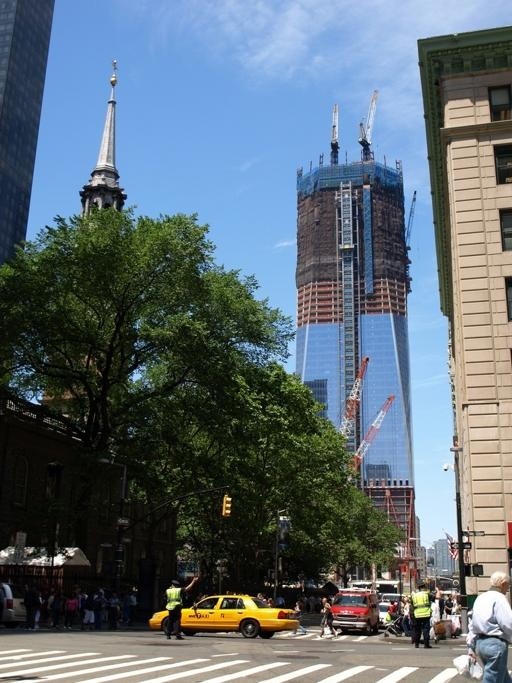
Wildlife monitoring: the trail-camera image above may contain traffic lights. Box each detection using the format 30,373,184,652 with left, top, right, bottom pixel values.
221,494,233,518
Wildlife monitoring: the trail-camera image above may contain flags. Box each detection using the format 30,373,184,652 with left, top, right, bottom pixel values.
447,535,459,560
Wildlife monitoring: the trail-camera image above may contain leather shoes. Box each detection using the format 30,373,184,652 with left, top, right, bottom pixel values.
167,636,184,640
416,645,431,648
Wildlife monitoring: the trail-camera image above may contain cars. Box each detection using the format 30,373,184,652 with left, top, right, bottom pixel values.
147,593,300,639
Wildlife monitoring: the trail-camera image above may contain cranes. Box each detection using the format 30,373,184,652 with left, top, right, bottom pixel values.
357,90,379,150
331,103,339,149
346,396,394,476
337,357,370,451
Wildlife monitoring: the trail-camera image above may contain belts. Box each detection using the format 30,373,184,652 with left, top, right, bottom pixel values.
480,634,500,639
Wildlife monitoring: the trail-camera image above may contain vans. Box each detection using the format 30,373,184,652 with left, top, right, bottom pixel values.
0,580,28,628
329,590,405,636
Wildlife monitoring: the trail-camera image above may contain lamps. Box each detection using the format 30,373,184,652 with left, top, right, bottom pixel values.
443,463,454,472
140,551,146,559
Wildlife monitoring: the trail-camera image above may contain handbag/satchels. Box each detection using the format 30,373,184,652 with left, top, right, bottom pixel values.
386,613,391,621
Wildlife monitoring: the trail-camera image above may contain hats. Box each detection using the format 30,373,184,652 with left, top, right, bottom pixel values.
172,580,179,587
415,581,425,588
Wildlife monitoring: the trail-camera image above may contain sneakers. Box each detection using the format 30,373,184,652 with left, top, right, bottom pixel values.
317,631,338,639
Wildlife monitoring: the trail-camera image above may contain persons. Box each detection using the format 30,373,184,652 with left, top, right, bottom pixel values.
193,590,285,608
465,570,512,683
319,597,339,638
0,584,5,625
387,579,462,649
297,593,335,614
291,601,308,634
23,584,138,630
164,576,199,640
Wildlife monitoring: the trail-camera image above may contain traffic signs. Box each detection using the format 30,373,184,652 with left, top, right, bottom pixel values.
451,542,471,549
